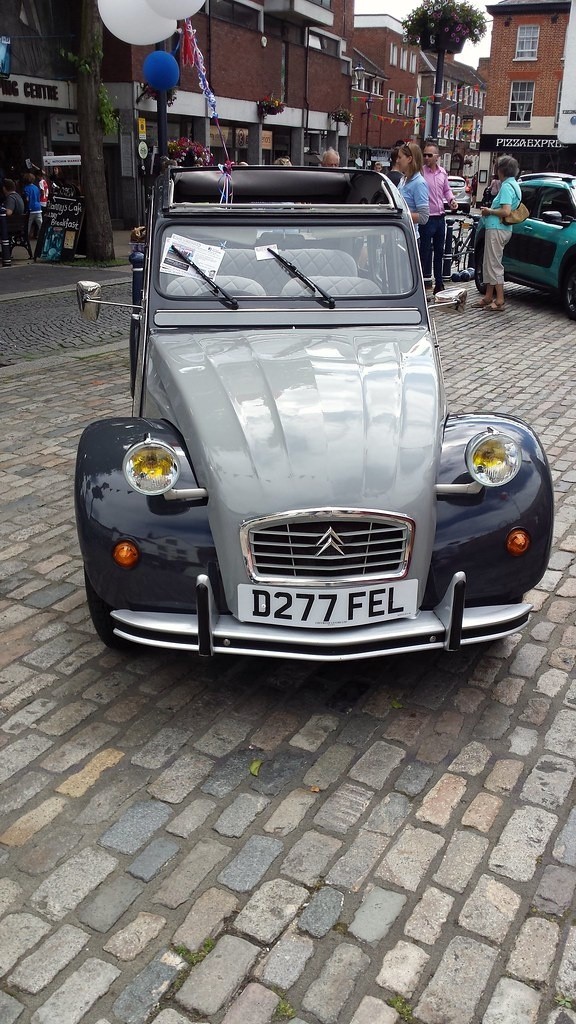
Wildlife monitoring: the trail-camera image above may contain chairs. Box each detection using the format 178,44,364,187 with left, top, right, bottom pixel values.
280,277,385,298
165,276,268,297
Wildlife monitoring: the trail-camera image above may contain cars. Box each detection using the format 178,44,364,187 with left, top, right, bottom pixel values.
74,163,554,660
443,176,471,213
474,172,576,321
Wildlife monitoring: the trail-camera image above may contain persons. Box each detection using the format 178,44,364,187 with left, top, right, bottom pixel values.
375,146,403,185
0,166,62,257
487,175,501,202
419,144,459,295
195,160,202,167
471,157,521,310
164,160,176,166
275,158,291,166
397,142,430,250
471,174,477,207
323,151,339,167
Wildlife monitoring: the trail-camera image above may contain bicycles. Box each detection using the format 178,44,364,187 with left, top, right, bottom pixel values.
451,212,481,271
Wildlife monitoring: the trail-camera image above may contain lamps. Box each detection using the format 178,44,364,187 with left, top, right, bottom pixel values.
362,92,374,114
349,61,365,89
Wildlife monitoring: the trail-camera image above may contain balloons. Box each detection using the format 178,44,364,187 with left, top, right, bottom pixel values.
143,51,180,91
98,0,177,45
147,0,205,20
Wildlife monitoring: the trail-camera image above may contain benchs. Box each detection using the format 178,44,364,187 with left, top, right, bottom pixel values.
5,210,35,261
162,250,357,281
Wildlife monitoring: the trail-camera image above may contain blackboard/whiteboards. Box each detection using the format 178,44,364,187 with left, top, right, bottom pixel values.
35,194,86,261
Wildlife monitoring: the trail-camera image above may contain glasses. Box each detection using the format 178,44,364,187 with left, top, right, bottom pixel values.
422,153,439,158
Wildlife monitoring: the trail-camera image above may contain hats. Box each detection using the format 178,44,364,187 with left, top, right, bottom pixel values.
397,140,404,145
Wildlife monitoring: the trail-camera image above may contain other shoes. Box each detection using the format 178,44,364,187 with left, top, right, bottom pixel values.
471,205,474,207
433,288,444,294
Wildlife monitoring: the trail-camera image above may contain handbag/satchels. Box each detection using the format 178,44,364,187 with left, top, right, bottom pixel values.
499,182,530,226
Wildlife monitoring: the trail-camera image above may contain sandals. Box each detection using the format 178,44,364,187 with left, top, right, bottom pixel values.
482,302,505,311
471,299,492,307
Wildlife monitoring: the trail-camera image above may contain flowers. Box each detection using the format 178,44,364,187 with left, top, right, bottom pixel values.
402,0,487,48
158,137,217,166
332,109,353,125
255,96,285,115
